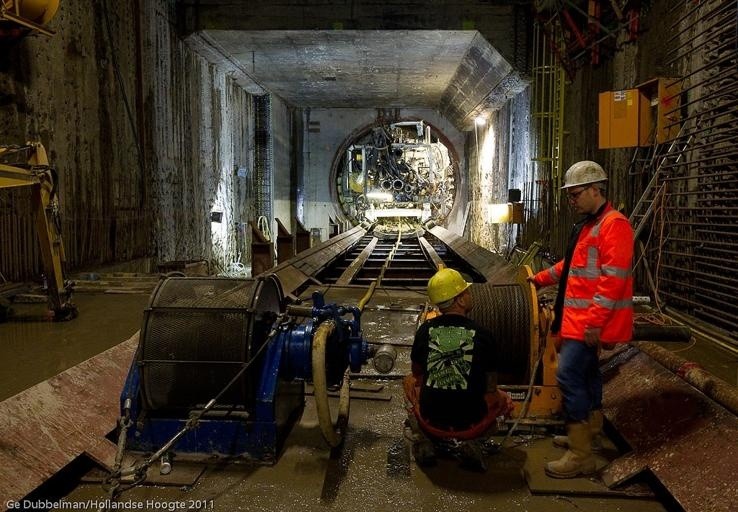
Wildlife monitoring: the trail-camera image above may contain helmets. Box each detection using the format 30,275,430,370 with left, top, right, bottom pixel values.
427,264,473,304
557,160,609,191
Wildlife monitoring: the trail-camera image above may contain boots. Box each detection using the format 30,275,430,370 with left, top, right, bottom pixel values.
408,415,438,465
544,422,597,479
552,409,604,453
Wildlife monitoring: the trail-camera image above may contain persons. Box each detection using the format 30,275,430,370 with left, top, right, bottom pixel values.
399,265,512,469
526,159,637,479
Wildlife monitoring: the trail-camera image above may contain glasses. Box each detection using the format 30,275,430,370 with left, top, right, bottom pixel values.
566,185,590,201
